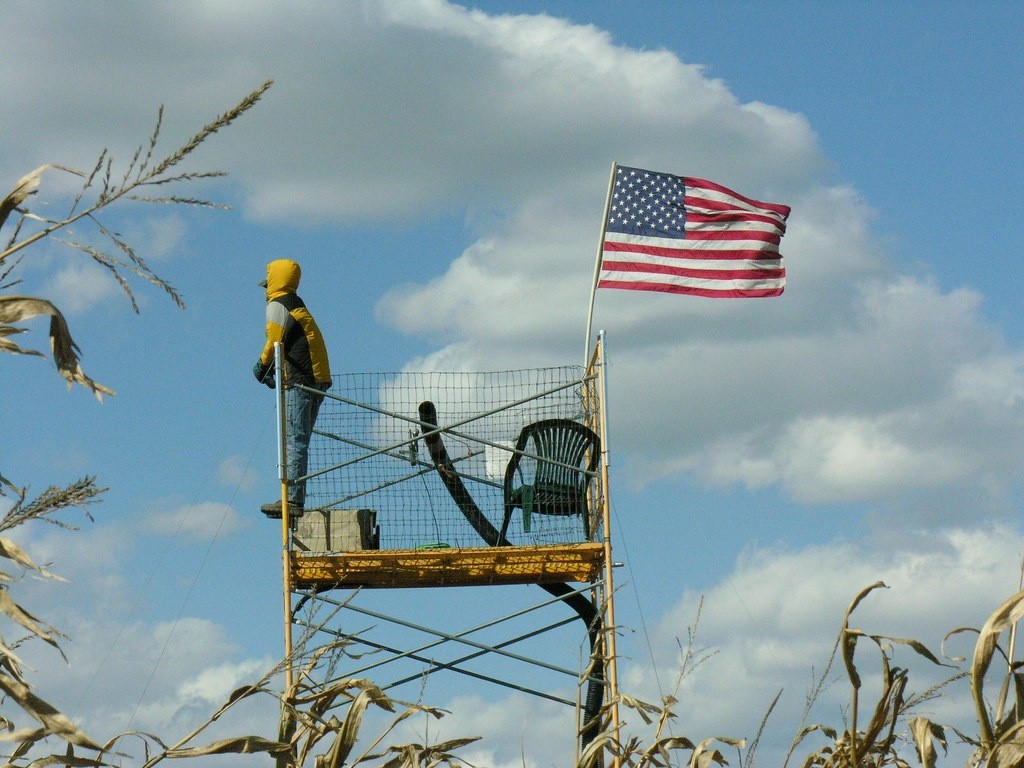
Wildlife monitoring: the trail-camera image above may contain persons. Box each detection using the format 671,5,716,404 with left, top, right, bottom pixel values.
254,259,332,519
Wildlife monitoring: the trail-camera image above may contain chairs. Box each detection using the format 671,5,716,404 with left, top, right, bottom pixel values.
494,418,603,546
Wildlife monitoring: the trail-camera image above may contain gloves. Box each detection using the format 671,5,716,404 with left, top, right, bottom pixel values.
265,370,276,389
253,357,269,383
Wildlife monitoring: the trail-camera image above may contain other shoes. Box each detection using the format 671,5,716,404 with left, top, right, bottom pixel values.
261,500,303,518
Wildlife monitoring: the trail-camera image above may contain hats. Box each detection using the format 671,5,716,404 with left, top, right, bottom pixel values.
257,279,268,286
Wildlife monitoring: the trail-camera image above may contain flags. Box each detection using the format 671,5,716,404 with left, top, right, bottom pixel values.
596,163,791,298
